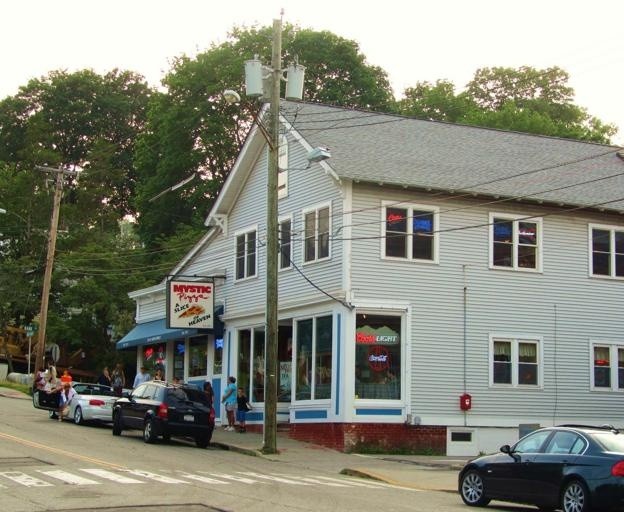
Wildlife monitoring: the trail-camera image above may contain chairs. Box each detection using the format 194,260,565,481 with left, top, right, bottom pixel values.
553,437,578,454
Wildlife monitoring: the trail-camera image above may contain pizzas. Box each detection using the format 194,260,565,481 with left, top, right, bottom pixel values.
179,304,205,318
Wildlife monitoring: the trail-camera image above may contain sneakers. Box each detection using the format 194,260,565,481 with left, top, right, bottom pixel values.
223,425,248,434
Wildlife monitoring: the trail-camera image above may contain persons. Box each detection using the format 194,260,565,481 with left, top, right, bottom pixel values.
31,360,255,434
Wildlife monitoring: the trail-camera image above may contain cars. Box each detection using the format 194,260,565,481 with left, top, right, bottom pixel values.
32,382,120,425
458,424,624,512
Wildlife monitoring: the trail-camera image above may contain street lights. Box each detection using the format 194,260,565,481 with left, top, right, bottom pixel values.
0,207,58,392
223,88,330,452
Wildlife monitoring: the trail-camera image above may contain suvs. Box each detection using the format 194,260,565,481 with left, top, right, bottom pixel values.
112,381,215,449
